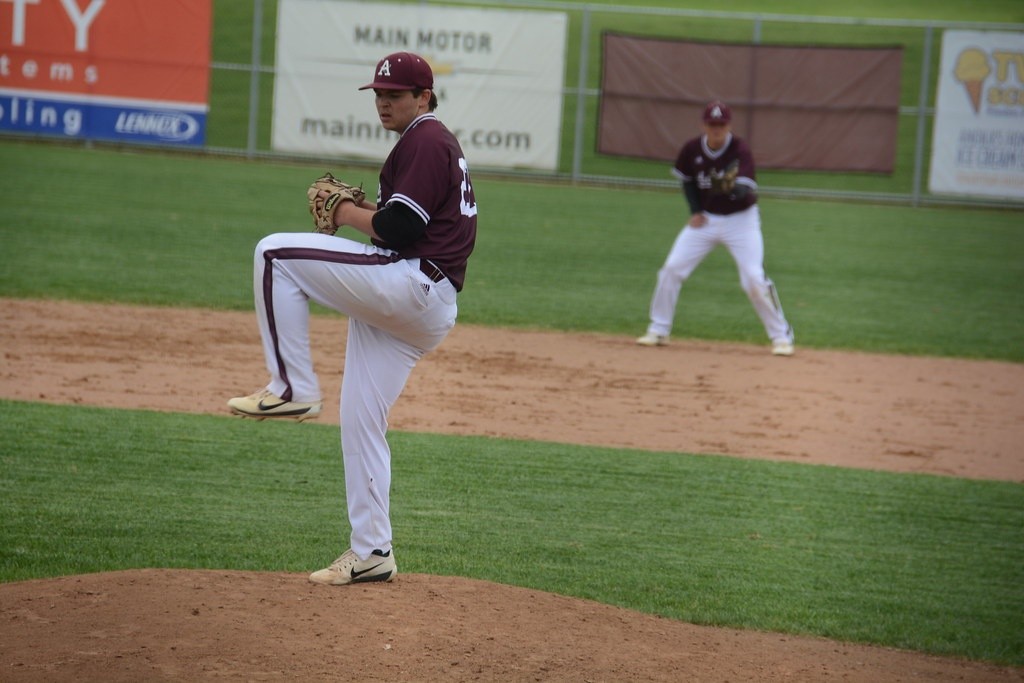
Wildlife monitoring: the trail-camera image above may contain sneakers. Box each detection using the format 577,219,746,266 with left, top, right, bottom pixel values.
772,342,793,356
226,388,320,423
635,332,670,346
309,549,397,586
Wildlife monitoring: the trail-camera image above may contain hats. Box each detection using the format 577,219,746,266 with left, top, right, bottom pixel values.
359,52,434,90
703,101,731,124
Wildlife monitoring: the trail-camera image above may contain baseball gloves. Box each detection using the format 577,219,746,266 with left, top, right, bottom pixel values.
308,176,365,235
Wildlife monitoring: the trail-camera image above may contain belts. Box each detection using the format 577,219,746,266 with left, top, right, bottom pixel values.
419,259,444,283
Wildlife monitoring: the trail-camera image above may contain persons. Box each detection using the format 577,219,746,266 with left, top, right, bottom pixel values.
636,102,796,355
228,51,478,585
